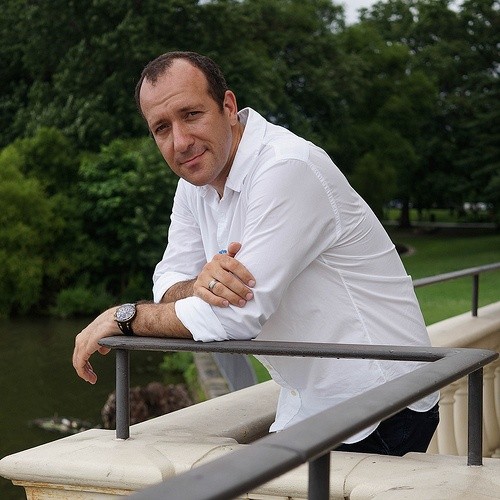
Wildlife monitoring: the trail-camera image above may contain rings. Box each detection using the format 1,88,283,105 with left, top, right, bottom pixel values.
208,279,218,291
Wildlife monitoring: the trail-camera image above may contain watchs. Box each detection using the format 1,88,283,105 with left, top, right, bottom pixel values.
114,303,138,337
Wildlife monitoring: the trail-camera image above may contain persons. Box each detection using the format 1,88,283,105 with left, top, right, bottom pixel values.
72,51,441,457
